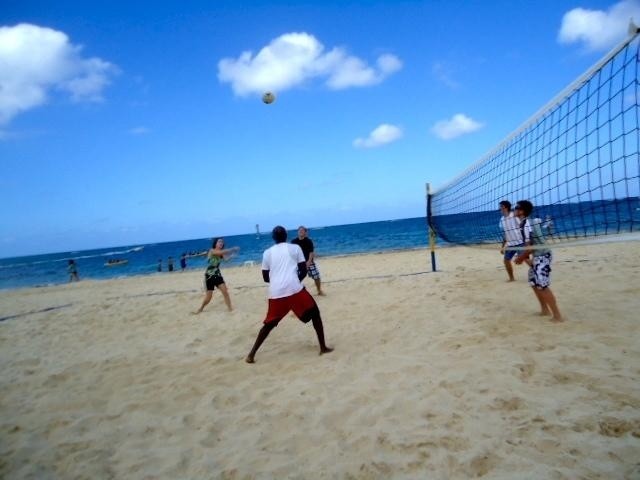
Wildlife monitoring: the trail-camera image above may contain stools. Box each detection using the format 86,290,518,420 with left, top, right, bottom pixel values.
243,260,256,270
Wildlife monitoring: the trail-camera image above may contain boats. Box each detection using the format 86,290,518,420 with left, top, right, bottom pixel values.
185,251,208,258
103,260,128,266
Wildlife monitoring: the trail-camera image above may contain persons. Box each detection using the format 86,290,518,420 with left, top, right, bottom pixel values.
189,237,242,316
290,226,327,297
496,200,532,283
243,227,335,364
66,260,79,283
167,257,173,272
180,253,187,273
156,259,162,272
511,200,565,322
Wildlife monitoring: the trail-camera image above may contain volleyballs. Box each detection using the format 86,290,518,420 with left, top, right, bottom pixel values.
261,91,275,104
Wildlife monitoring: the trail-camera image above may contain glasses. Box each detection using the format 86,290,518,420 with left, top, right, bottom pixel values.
514,206,522,210
